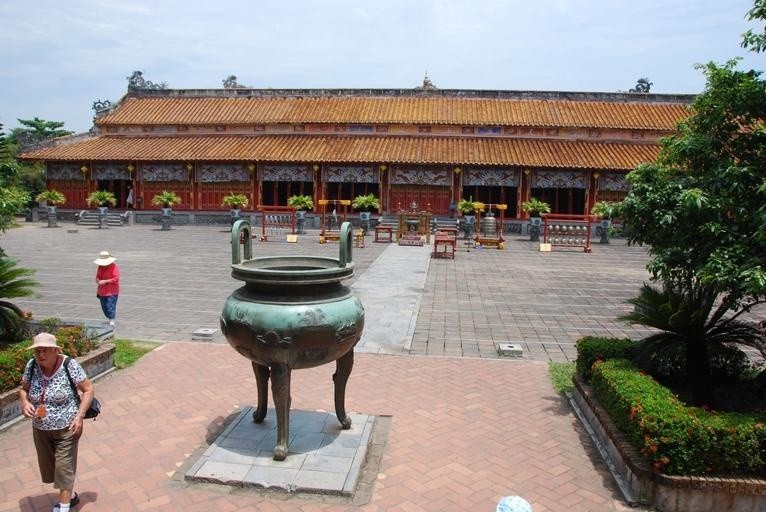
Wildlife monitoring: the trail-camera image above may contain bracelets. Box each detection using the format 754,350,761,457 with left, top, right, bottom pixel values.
76,408,86,418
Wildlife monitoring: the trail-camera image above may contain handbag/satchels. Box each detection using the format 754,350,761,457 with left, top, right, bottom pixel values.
84,397,101,419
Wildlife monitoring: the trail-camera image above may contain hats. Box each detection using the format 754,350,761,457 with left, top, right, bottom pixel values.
94,251,116,266
26,333,60,350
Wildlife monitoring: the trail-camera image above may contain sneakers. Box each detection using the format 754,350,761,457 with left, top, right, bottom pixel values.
109,319,115,325
52,492,79,508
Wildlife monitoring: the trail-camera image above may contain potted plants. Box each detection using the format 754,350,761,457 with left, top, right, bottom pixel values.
590,200,621,230
85,189,117,214
220,191,249,218
151,189,181,217
521,196,552,228
286,194,314,220
351,193,380,221
35,189,65,212
457,195,486,225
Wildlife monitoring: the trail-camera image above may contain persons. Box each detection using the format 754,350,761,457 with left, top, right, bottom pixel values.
93,250,120,326
17,331,95,511
125,184,133,210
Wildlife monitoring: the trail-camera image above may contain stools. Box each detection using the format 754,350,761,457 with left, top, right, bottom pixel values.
375,226,392,243
434,227,457,259
